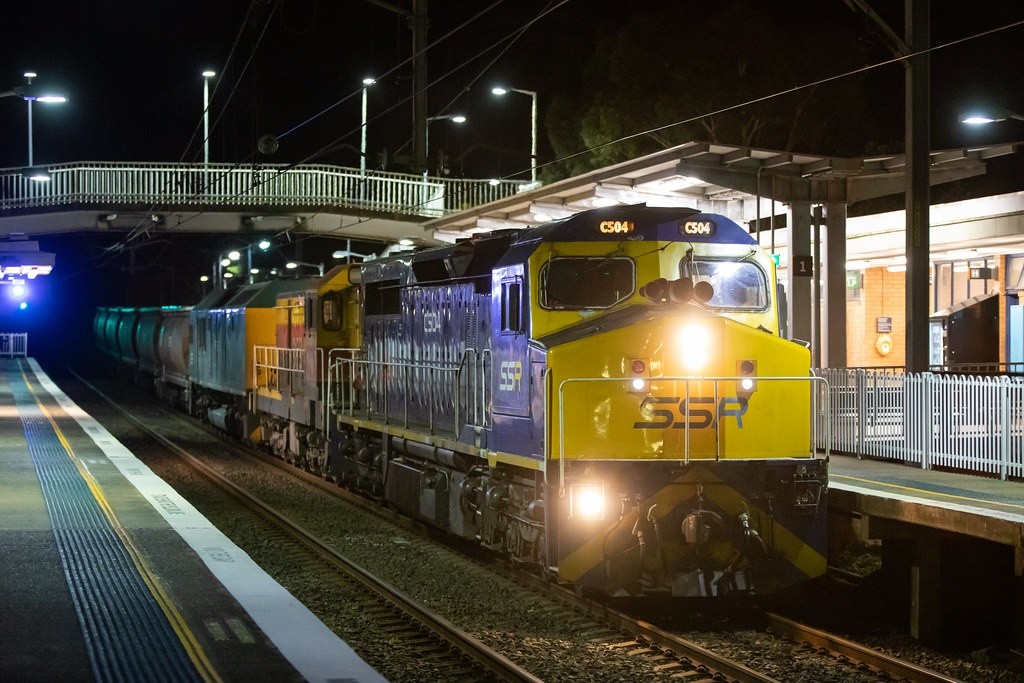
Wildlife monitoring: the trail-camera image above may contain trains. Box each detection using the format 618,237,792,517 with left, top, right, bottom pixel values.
83,202,830,600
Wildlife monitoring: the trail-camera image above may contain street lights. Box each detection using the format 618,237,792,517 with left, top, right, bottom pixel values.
286,262,323,277
200,70,217,204
491,84,538,187
421,115,466,214
362,77,376,206
24,70,37,204
